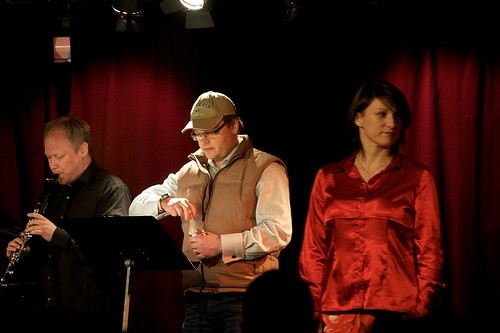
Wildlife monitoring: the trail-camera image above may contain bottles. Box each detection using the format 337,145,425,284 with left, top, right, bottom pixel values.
187,211,213,263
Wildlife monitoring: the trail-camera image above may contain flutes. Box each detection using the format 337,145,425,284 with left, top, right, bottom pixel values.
0,174,58,287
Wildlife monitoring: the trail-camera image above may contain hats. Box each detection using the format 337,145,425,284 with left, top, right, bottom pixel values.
181,90,237,133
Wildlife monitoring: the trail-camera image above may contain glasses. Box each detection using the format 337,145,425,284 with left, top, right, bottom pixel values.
190,117,231,141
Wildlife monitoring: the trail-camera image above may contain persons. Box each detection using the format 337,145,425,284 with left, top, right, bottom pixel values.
295,79,446,333
5,115,133,333
128,90,292,333
